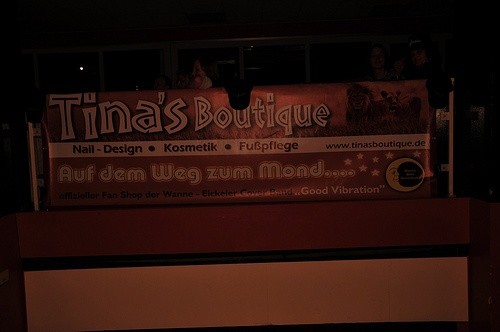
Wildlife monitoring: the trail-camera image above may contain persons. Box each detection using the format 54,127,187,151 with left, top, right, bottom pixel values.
369,43,390,81
175,69,190,88
154,75,172,90
190,57,212,88
188,75,203,89
387,55,405,81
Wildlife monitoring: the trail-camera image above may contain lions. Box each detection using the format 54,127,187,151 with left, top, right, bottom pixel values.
343,84,387,132
381,89,422,128
